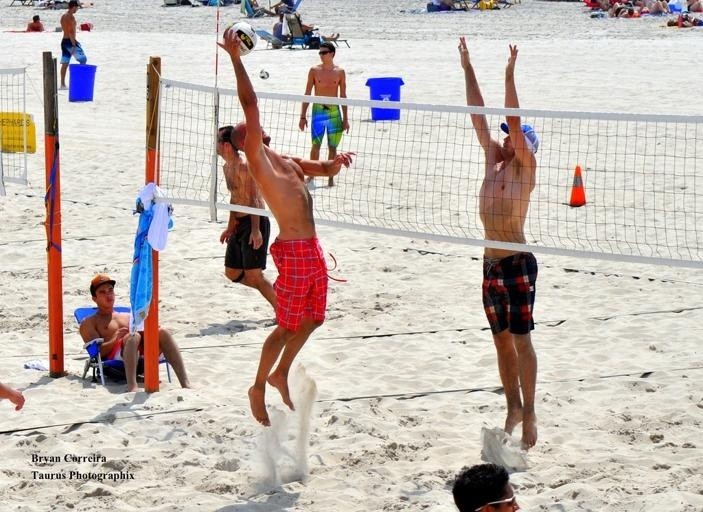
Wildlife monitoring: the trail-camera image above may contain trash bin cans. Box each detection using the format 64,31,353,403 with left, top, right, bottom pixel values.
68,64,96,103
365,78,405,122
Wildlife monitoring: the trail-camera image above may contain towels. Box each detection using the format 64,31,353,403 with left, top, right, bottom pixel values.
140,182,169,250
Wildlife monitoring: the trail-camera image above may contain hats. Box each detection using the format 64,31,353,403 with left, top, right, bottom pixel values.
67,0,85,10
500,121,539,156
89,275,117,292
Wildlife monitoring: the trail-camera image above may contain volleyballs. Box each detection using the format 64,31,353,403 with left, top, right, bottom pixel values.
259,69,269,79
222,22,257,56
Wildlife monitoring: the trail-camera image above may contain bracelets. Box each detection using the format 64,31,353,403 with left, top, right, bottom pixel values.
300,117,306,119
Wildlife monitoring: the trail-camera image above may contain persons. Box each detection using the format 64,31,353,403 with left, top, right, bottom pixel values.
215,125,278,310
458,37,539,451
80,273,192,392
248,0,273,18
273,13,291,42
270,1,293,13
454,464,518,511
586,0,700,18
217,27,356,426
295,13,340,41
299,43,350,187
60,0,87,90
27,15,44,32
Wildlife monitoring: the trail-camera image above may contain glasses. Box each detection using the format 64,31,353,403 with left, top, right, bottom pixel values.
474,495,520,512
318,50,336,56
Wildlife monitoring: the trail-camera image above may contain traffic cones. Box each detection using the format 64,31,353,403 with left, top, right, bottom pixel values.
569,164,586,207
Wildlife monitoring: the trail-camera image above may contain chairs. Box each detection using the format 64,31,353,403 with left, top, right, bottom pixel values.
241,1,264,18
287,16,350,49
257,31,304,49
278,0,301,16
75,308,171,386
